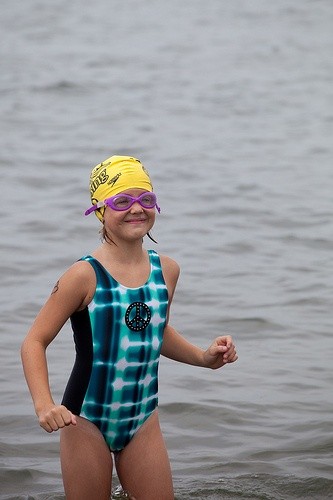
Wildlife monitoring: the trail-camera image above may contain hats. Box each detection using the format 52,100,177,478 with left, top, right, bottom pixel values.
89,155,154,225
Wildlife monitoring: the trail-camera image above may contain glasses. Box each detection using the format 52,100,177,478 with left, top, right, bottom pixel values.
84,191,157,216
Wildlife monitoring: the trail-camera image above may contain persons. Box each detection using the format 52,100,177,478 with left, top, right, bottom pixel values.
20,155,238,500
